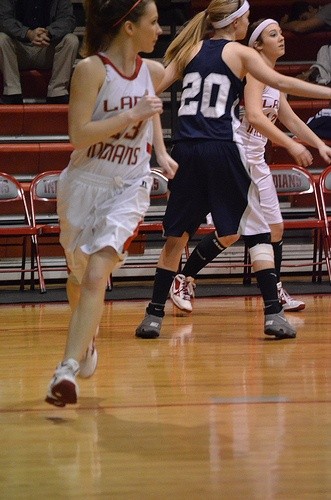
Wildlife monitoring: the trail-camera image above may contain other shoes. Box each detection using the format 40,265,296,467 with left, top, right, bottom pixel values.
46,93,70,104
0,93,25,106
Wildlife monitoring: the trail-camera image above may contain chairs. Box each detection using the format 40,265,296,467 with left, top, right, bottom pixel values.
120,171,184,318
29,171,113,292
249,164,331,286
315,165,331,286
186,212,248,289
0,172,46,293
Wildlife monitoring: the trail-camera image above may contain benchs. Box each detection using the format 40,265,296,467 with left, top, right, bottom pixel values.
0,23,331,213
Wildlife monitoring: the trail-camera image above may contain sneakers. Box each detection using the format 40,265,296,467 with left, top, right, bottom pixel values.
169,274,196,313
79,325,99,378
44,358,82,408
276,281,306,311
135,307,165,339
263,302,296,338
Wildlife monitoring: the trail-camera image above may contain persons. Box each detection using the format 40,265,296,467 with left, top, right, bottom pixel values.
169,17,331,313
278,0,331,34
43,0,179,408
0,0,79,103
136,0,331,339
